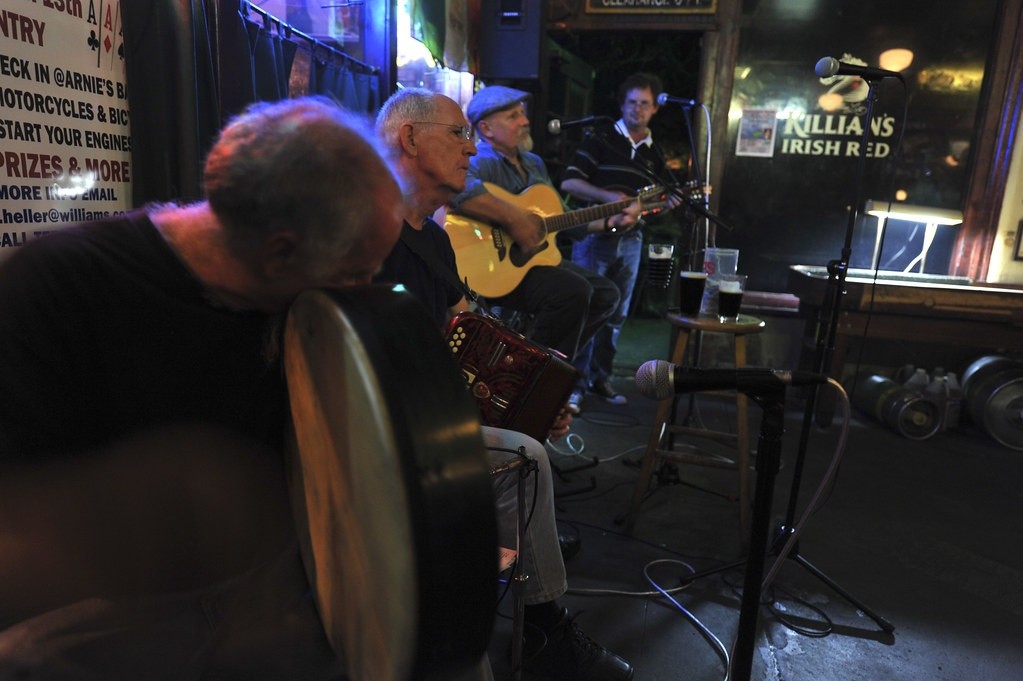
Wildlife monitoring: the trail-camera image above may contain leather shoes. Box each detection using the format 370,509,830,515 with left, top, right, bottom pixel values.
556,531,580,556
520,604,634,681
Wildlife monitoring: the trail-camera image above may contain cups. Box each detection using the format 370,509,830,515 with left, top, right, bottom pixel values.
699,248,739,315
680,270,708,318
648,244,674,288
719,274,748,323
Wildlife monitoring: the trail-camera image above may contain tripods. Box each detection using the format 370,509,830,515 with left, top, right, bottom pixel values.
613,205,753,524
680,81,895,634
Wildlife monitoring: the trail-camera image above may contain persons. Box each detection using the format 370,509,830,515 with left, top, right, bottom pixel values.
371,87,631,681
442,87,623,365
561,72,684,415
0,95,408,681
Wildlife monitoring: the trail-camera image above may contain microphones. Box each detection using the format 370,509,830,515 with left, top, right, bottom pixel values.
634,360,825,400
815,56,902,79
547,115,595,134
657,93,695,106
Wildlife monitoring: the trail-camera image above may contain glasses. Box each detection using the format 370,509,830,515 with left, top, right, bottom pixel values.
412,121,472,143
624,101,654,111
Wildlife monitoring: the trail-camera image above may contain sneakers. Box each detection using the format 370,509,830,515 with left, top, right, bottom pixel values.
584,378,627,405
567,391,584,414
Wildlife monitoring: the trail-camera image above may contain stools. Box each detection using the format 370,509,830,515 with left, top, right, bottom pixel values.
625,313,764,546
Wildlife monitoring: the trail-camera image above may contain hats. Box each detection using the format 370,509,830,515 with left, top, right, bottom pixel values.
467,86,532,124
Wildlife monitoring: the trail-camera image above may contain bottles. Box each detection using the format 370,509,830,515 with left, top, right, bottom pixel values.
894,364,963,431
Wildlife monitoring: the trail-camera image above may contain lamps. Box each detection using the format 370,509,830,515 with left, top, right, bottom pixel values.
864,200,965,272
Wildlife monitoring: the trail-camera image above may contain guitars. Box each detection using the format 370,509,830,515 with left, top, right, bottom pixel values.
586,180,713,238
443,183,669,300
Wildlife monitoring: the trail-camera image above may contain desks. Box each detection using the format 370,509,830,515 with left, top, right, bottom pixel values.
788,265,1023,430
